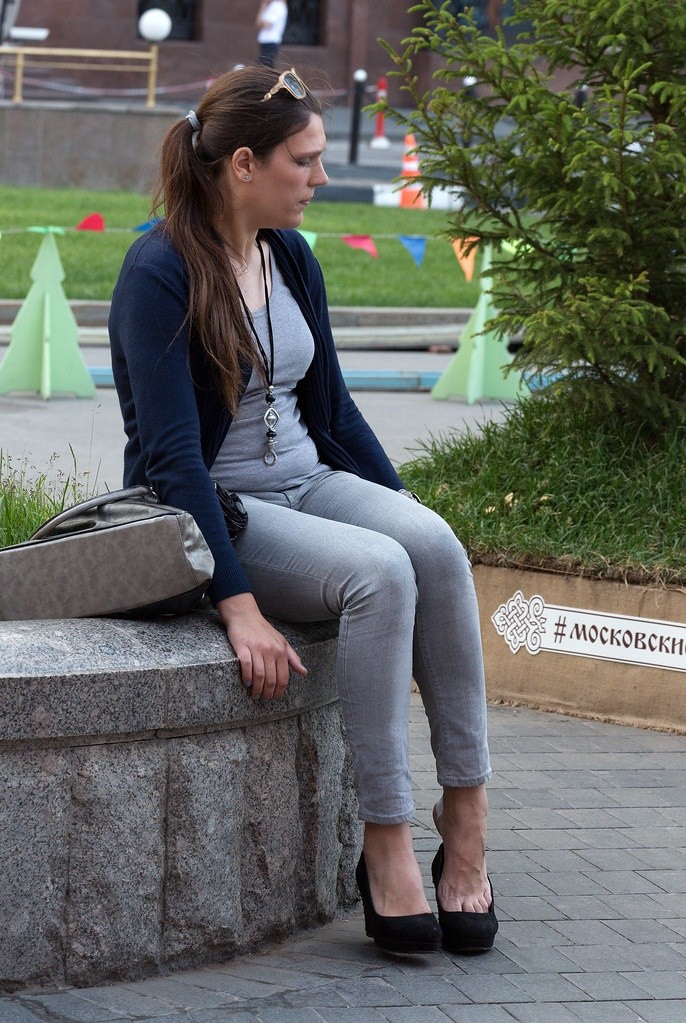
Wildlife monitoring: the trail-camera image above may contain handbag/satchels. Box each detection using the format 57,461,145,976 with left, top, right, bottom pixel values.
0,482,217,617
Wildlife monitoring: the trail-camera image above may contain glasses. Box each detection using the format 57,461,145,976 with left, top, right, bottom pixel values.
260,66,312,102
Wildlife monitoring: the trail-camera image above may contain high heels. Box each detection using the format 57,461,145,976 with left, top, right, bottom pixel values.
430,841,499,951
354,849,442,955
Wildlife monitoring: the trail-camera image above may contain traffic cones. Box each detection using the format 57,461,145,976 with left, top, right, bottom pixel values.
400,130,425,209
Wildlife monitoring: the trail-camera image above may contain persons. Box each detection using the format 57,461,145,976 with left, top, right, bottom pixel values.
109,66,498,954
255,0,287,68
